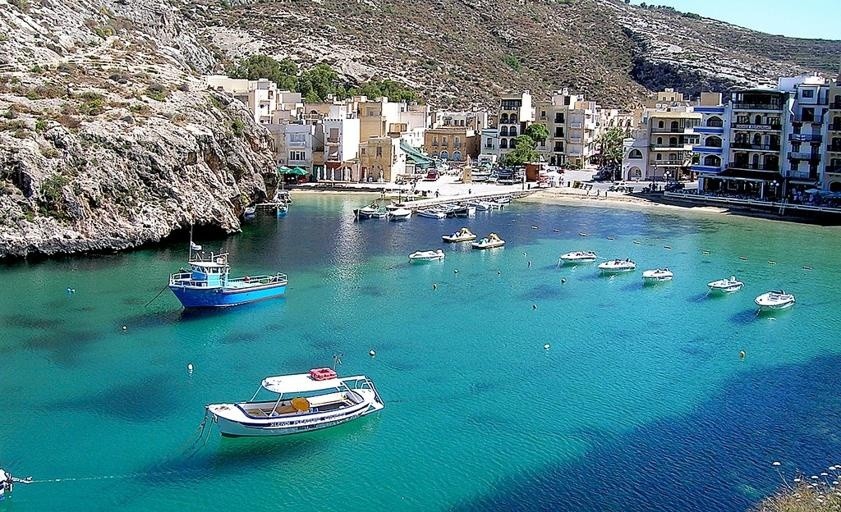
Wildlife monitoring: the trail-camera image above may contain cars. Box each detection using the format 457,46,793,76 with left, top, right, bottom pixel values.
609,181,628,190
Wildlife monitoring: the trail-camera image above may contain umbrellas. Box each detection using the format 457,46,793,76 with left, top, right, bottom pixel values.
278,166,292,174
288,167,309,180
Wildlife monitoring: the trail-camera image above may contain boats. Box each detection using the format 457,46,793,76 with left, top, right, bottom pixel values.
598,259,636,272
417,194,512,218
353,206,411,220
754,289,795,307
409,250,444,260
560,251,596,263
168,219,288,307
205,368,385,436
642,268,673,281
442,228,505,248
707,276,744,291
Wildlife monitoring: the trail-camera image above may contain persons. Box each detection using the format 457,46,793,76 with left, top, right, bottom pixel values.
559,177,564,185
434,189,440,198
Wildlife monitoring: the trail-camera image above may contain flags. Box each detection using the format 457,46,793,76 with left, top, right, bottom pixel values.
191,242,202,251
